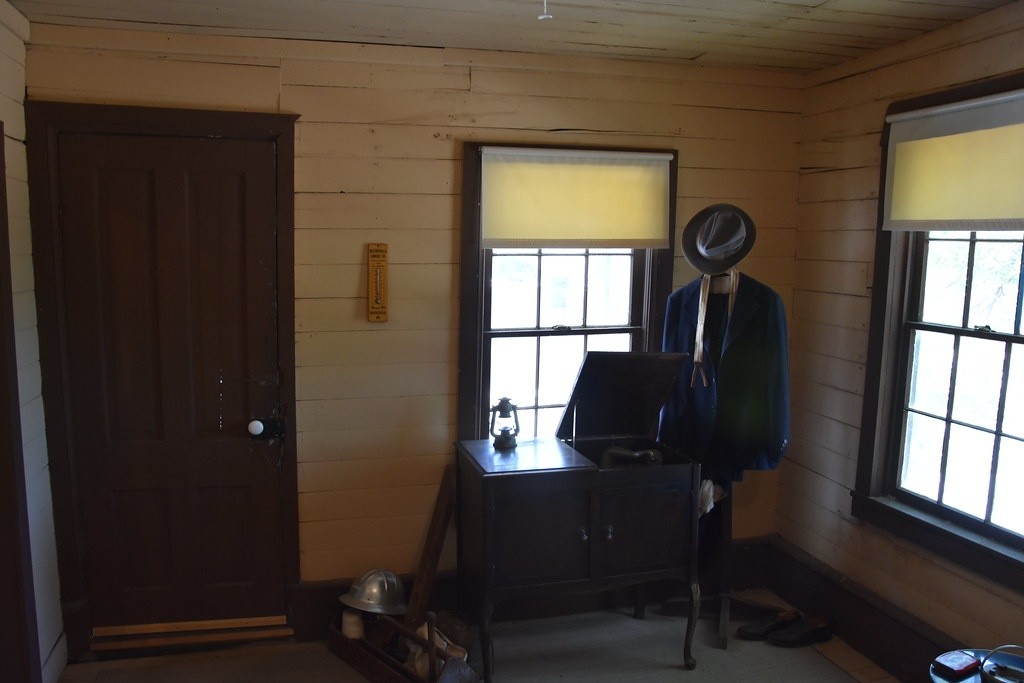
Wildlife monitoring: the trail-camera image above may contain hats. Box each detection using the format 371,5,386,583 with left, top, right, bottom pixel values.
681,202,757,276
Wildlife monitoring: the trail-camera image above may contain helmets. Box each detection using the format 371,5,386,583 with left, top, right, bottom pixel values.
339,568,408,614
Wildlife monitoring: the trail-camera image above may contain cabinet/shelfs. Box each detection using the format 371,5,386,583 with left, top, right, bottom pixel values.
452,351,702,683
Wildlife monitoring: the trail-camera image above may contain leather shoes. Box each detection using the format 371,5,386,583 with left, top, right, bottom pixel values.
737,608,802,640
767,615,832,647
